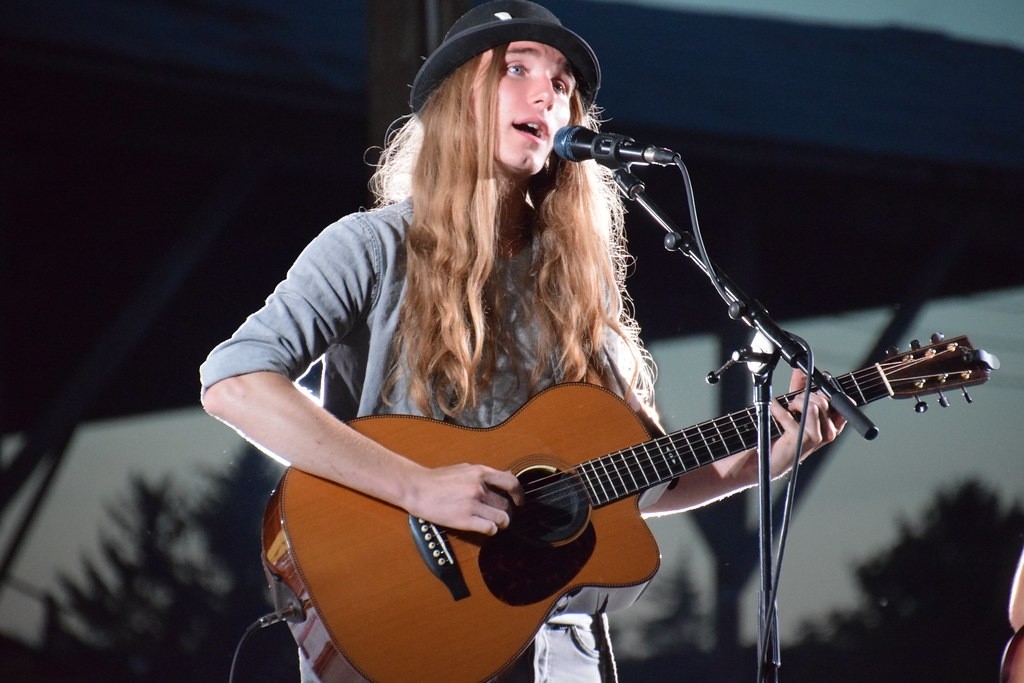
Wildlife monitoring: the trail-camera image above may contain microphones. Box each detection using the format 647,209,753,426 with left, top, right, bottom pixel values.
553,125,679,167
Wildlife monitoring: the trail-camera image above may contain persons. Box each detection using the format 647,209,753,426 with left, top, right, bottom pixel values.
199,0,849,682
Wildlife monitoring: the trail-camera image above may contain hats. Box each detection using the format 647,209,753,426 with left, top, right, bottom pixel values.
408,0,601,109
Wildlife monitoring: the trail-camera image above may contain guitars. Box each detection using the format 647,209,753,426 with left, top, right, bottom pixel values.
261,333,999,683
